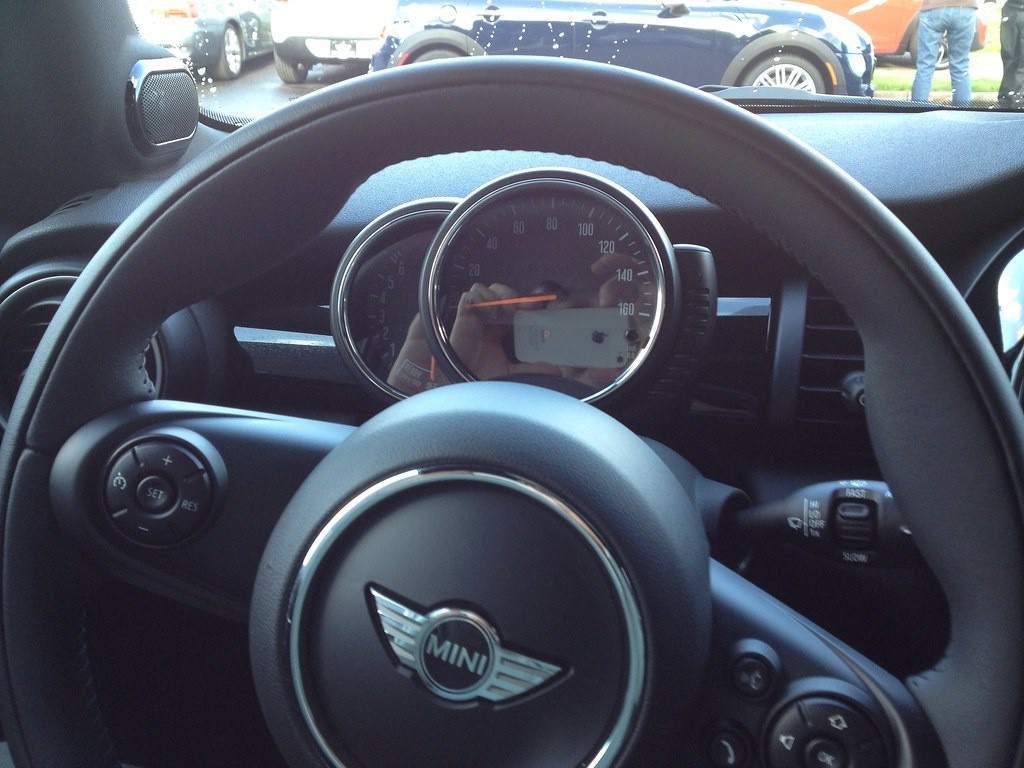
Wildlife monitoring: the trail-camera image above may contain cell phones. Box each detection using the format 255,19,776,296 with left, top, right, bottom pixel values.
513,307,628,368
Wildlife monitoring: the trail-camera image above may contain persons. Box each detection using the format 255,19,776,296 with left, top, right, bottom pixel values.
911,0,978,107
449,250,651,388
997,0,1024,100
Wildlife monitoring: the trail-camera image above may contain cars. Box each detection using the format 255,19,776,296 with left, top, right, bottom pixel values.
133,0,985,98
